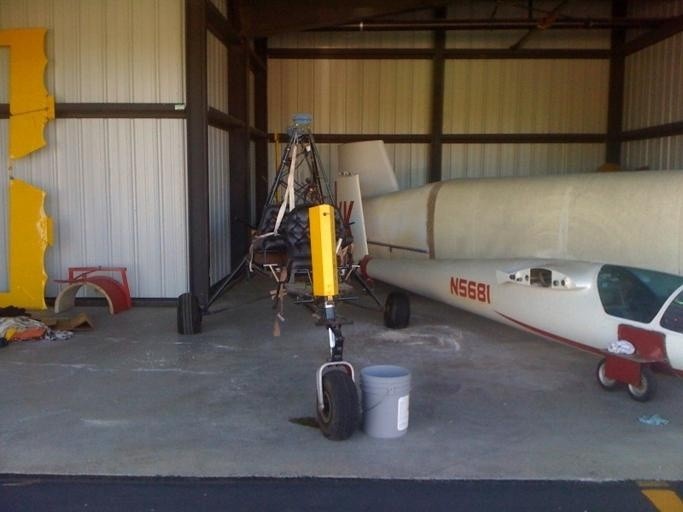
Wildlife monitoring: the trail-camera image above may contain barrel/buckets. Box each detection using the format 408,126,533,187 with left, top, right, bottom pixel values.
360,363,412,439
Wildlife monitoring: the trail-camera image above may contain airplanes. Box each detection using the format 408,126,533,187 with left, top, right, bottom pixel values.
337,141,683,277
